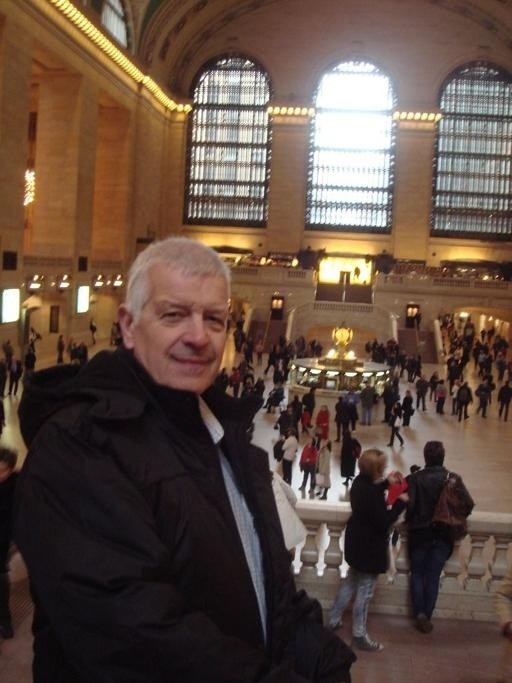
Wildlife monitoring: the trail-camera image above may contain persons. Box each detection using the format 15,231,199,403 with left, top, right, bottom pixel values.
13,237,358,682
0,314,511,654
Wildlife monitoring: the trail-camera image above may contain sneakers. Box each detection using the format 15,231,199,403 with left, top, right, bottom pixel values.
417,614,434,633
351,633,383,651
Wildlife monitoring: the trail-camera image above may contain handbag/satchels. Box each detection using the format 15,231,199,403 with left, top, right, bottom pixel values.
432,475,469,540
393,415,401,428
405,409,414,415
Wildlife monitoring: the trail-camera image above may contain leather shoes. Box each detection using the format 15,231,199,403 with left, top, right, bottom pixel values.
316,492,322,495
319,496,327,499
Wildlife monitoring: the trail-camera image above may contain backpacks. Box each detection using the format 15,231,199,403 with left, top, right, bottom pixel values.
351,438,362,458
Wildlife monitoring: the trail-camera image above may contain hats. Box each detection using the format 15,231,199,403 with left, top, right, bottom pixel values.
410,465,420,472
